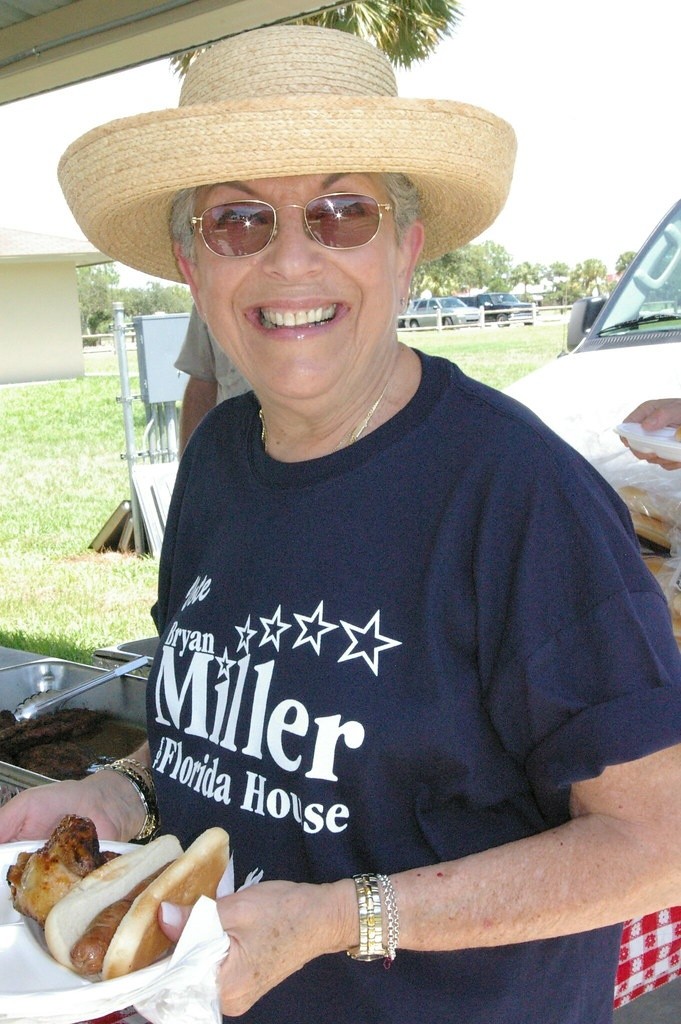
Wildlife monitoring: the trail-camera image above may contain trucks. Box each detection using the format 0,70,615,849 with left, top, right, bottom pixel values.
457,293,538,327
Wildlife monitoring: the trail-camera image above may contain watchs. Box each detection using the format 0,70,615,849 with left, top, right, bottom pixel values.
346,871,388,962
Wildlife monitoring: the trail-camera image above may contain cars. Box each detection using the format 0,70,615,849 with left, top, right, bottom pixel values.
405,296,480,330
398,315,406,329
108,323,135,334
498,198,681,460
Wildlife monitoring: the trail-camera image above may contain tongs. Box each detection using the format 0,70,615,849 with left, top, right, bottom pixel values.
15,658,150,723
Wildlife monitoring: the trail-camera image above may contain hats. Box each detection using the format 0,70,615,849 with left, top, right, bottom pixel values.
56,25,517,284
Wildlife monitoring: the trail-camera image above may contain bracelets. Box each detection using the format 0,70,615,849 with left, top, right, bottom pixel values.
116,757,160,825
94,761,156,841
379,873,400,962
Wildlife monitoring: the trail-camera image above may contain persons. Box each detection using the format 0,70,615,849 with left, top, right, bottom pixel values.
0,25,680,1024
618,397,680,473
174,303,254,461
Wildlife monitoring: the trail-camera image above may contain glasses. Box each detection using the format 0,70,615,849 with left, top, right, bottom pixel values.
191,192,393,257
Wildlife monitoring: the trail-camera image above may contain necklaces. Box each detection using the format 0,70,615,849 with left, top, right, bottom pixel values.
260,343,402,470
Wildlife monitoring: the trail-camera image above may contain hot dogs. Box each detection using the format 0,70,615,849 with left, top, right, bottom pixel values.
43,827,230,980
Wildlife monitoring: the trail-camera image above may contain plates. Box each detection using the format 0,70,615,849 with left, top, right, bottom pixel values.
614,418,681,461
0,838,209,1023
